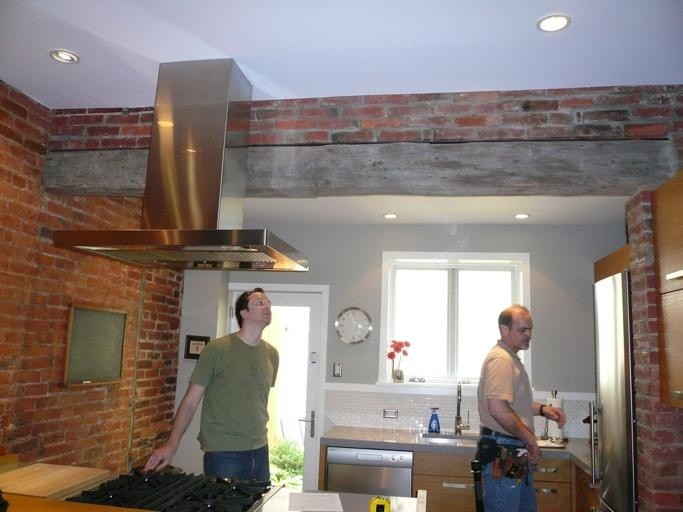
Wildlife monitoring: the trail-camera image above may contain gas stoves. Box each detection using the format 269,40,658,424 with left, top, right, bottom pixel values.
65,467,285,512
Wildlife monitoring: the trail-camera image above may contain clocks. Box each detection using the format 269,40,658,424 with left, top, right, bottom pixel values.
332,306,372,346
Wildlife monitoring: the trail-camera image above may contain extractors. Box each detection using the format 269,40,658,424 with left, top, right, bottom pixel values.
51,58,309,273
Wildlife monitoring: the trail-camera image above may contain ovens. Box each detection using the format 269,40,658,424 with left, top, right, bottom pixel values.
325,447,413,497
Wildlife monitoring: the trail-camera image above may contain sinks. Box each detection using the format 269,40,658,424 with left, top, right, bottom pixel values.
419,432,477,445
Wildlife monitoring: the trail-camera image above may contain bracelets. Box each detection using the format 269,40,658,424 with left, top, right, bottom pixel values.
540,404,547,416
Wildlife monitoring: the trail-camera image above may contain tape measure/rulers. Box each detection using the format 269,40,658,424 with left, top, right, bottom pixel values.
368,496,391,512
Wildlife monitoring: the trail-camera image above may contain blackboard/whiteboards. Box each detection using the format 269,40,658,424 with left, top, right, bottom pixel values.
63,304,128,388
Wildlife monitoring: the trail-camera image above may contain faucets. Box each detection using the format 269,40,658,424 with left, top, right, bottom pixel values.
454,384,471,434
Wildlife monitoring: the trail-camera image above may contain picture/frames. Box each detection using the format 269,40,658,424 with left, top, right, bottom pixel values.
183,334,211,360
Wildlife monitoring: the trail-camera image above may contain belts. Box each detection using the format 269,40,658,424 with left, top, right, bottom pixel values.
480,427,522,441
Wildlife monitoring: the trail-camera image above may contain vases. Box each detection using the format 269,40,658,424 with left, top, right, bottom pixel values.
393,369,403,383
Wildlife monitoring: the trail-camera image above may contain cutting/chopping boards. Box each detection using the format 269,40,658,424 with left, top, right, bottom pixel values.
1,464,112,501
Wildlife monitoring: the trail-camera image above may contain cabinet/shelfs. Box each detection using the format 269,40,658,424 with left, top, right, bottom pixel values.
573,465,600,511
412,451,476,512
647,169,682,409
521,455,572,512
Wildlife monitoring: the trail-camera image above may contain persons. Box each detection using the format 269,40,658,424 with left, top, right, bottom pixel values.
473,303,566,512
142,287,279,481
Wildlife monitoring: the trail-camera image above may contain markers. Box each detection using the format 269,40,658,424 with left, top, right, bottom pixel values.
81,381,91,383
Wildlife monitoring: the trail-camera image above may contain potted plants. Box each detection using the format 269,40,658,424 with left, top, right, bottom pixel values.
386,339,411,369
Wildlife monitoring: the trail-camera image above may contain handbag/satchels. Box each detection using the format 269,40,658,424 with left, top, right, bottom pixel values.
477,438,498,464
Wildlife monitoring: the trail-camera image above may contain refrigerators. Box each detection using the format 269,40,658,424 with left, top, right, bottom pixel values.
588,269,637,512
